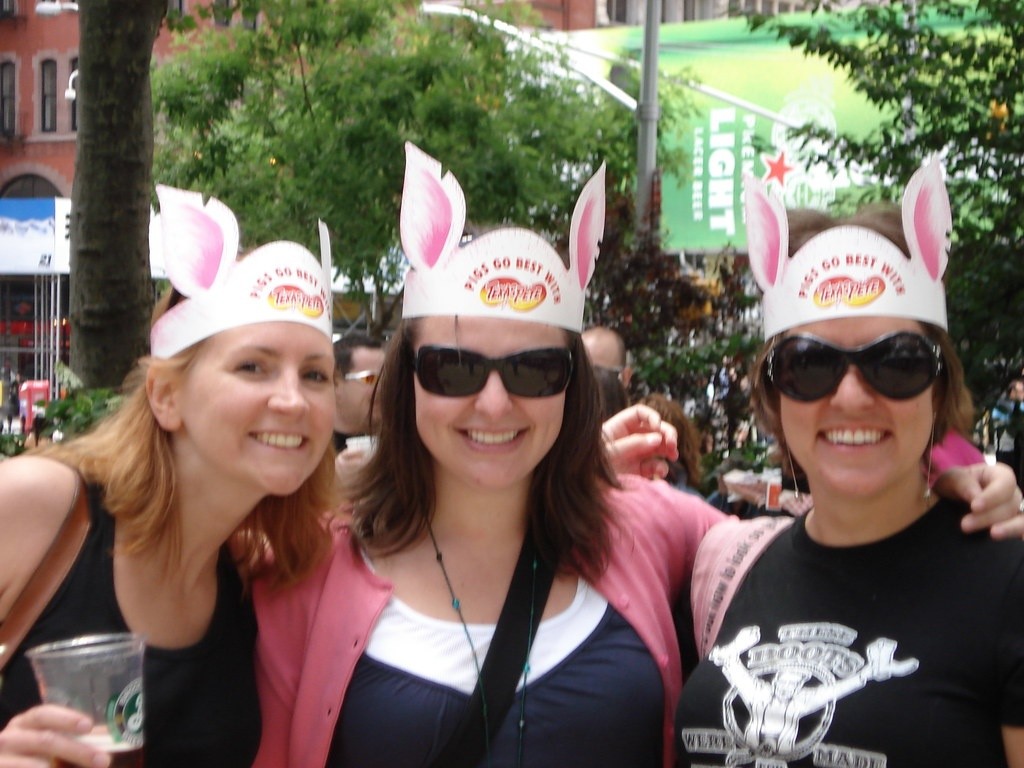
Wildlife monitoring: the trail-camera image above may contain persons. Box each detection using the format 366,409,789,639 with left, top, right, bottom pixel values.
252,137,1024,767
328,325,701,481
674,157,1024,767
687,358,1024,461
0,183,679,768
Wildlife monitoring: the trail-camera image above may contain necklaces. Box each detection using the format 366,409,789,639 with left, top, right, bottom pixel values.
428,515,541,767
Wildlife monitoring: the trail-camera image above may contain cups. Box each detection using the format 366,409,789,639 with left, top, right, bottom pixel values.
25,632,147,752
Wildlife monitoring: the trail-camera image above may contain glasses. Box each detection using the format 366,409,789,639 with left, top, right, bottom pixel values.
766,330,940,401
413,345,574,398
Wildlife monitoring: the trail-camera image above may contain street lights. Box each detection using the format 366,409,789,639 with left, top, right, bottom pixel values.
416,0,662,259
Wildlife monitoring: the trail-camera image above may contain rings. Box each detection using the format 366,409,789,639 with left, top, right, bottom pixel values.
1019,501,1024,512
